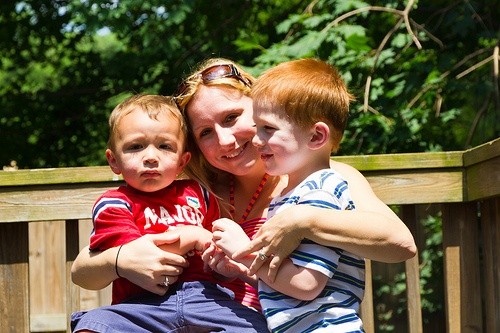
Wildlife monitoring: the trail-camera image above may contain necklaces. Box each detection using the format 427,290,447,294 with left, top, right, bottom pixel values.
229,174,267,226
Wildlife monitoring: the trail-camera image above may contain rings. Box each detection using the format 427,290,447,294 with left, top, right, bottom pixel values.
259,251,267,261
164,275,169,287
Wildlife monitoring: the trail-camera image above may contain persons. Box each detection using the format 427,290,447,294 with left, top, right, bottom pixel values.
69,95,271,333
201,59,367,333
71,61,418,333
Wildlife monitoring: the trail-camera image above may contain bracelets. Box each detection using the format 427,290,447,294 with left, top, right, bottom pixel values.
115,243,124,278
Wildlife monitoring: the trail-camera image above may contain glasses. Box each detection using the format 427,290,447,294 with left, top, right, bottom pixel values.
174,64,252,97
